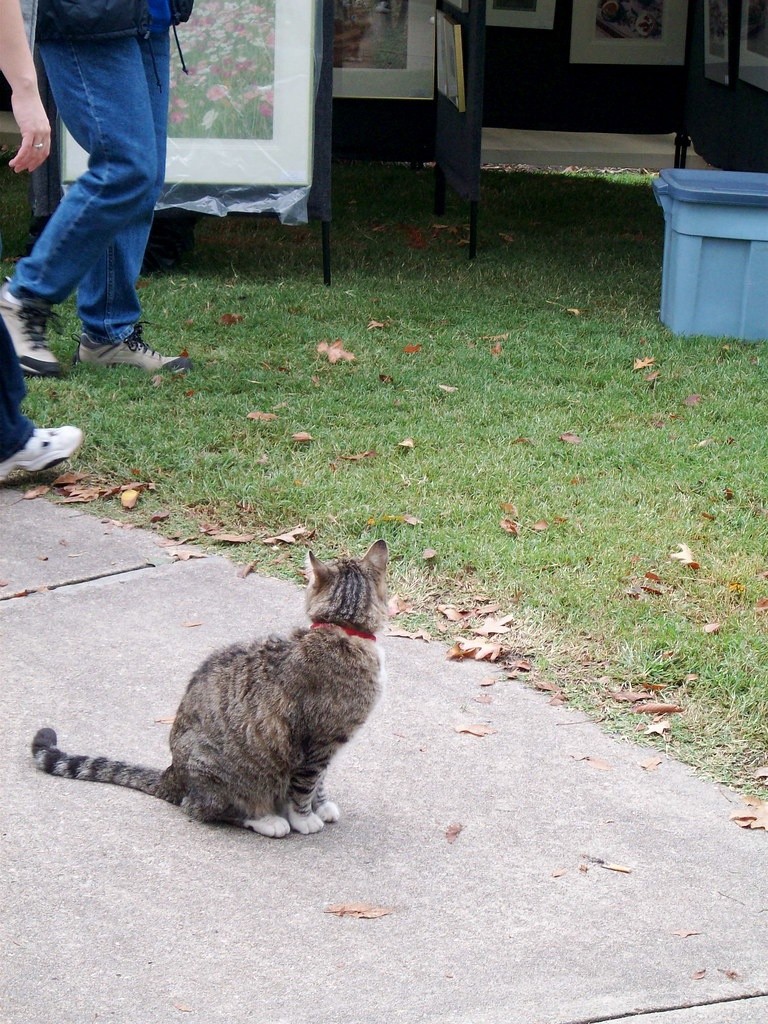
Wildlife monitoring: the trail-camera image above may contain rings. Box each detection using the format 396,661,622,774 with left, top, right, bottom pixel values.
33,143,44,148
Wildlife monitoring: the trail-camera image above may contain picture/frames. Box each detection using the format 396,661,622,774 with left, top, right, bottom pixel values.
485,0,556,30
703,1,729,86
332,0,469,113
569,0,689,66
739,0,768,91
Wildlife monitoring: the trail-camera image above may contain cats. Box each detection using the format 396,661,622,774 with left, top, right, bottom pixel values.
31,540,388,838
334,0,374,68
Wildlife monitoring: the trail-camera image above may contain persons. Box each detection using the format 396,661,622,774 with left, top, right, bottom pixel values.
0,0,194,376
0,0,84,483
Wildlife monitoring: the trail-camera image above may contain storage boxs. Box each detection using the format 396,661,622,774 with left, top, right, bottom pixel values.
652,169,768,342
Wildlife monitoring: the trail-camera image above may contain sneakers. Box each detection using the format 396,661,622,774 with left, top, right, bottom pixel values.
71,321,193,375
0,276,66,377
0,426,81,483
